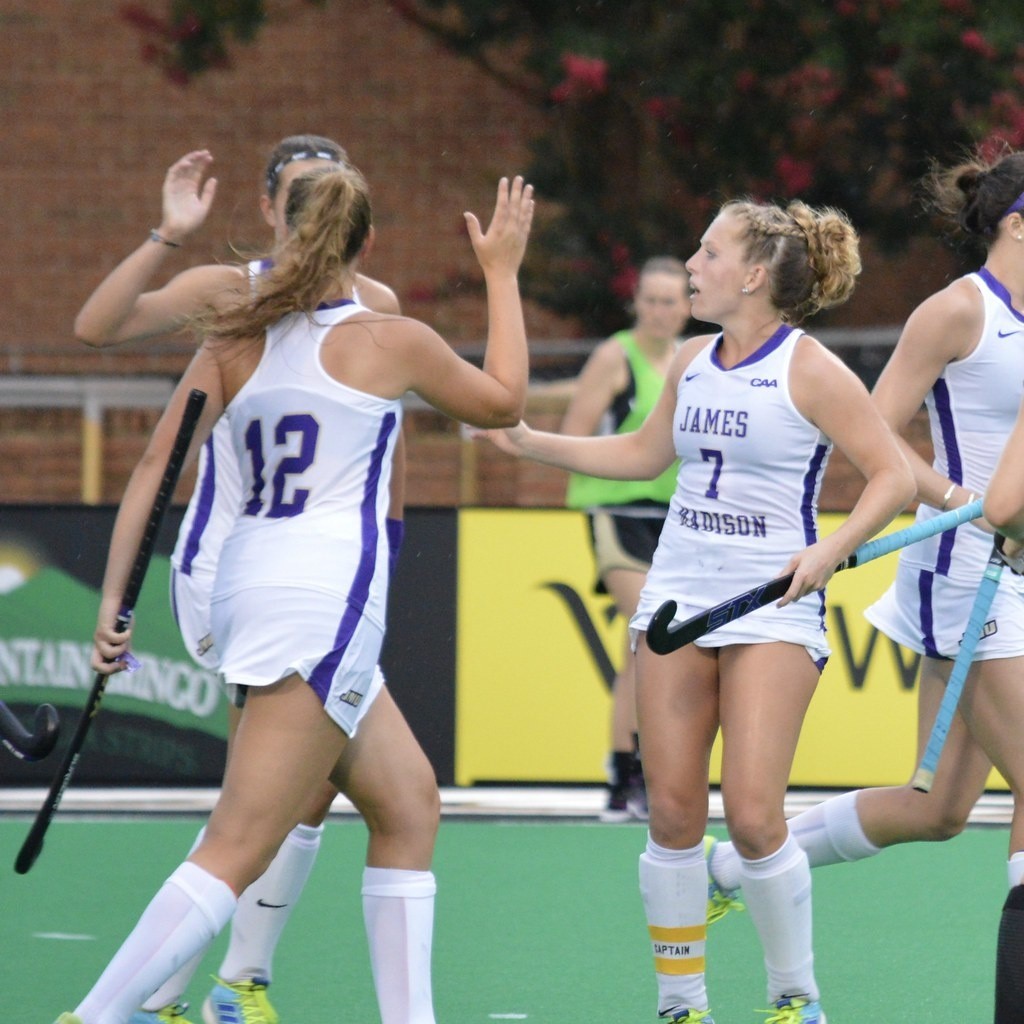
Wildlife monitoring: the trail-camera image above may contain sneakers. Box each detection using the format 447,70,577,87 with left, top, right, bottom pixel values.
658,1009,714,1024
127,1003,194,1024
702,835,745,926
52,1011,82,1024
201,973,280,1024
753,992,826,1024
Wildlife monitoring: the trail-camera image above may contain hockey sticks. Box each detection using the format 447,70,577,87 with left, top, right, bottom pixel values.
908,530,1008,798
644,493,987,658
13,387,210,876
0,697,62,761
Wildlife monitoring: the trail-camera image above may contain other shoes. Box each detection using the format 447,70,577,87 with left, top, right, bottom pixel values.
627,795,651,820
599,800,632,822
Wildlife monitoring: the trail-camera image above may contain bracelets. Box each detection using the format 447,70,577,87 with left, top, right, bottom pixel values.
939,483,975,516
150,229,180,250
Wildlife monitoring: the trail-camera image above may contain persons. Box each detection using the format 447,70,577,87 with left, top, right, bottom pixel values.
49,132,695,1024
475,197,918,1024
700,149,1024,893
